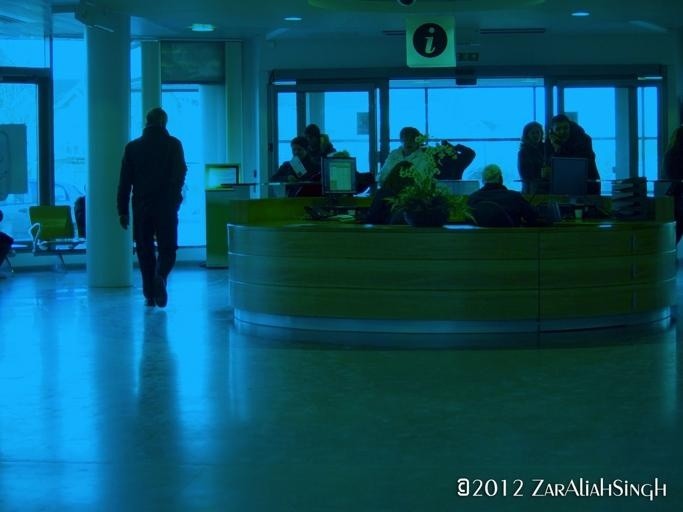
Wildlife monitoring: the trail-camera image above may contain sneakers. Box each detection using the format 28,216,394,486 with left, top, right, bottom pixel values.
144,277,166,308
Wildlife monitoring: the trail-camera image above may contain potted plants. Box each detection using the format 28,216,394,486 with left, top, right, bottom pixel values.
388,135,462,225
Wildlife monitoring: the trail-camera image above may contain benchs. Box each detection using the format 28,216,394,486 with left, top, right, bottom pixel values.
28,205,158,274
0,244,15,274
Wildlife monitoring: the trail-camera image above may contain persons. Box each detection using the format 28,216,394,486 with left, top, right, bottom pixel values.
303,124,335,161
116,107,188,308
516,121,553,195
378,126,434,193
663,126,682,245
266,136,320,197
465,164,557,226
544,113,601,196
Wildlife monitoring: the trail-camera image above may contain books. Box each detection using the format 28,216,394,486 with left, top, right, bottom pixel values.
612,182,640,217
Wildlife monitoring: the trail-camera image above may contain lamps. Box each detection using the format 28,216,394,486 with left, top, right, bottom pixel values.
570,10,590,17
377,13,549,36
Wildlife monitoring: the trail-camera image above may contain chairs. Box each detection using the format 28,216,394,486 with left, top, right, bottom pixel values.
381,195,408,225
472,201,514,227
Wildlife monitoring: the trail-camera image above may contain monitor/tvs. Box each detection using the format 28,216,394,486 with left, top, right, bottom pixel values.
550,157,586,196
437,180,479,196
319,156,358,208
